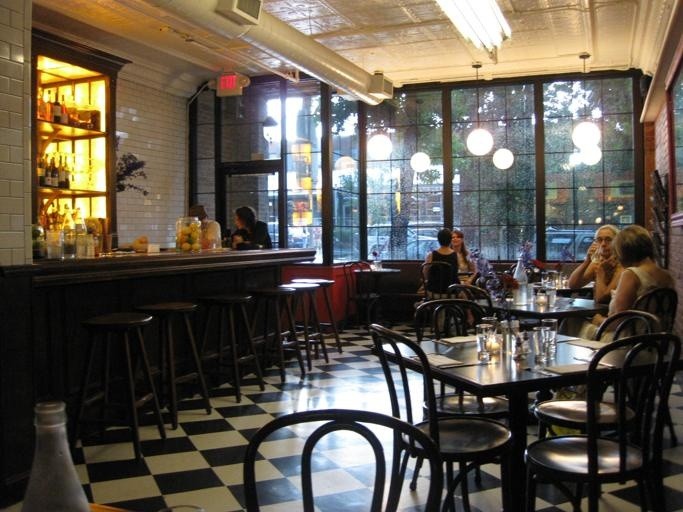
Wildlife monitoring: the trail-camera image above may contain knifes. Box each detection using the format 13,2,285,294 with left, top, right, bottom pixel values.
571,356,616,368
438,362,489,369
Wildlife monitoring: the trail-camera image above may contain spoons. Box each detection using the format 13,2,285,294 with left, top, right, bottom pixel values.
517,362,550,376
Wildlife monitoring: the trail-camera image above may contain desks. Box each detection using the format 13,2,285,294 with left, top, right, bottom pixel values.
2,495,125,512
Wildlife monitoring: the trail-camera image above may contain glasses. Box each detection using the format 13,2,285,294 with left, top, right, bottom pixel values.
597,237,612,243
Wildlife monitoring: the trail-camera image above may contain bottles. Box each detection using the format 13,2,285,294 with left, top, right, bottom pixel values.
512,258,528,305
37,151,70,188
62,204,87,258
496,254,502,271
39,201,61,230
37,88,80,128
32,225,47,261
174,216,202,254
18,398,91,512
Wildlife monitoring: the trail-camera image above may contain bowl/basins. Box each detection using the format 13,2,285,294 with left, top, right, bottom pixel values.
132,243,149,253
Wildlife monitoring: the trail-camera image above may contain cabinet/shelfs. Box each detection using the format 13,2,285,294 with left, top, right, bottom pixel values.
30,50,113,250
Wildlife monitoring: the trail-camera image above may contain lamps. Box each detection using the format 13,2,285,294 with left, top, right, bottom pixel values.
571,51,605,152
429,1,513,49
463,63,493,157
221,114,277,128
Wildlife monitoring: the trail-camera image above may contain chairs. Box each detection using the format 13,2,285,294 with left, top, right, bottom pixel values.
237,407,445,512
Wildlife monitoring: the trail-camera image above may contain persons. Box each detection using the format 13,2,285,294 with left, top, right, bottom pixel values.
188,205,222,250
422,228,458,333
554,224,675,352
448,230,477,326
229,205,272,251
567,223,625,306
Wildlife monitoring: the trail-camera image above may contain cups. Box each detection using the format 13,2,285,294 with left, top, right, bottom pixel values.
589,246,604,265
369,260,383,270
545,283,556,306
540,270,568,287
475,315,558,364
536,293,548,306
47,230,113,259
